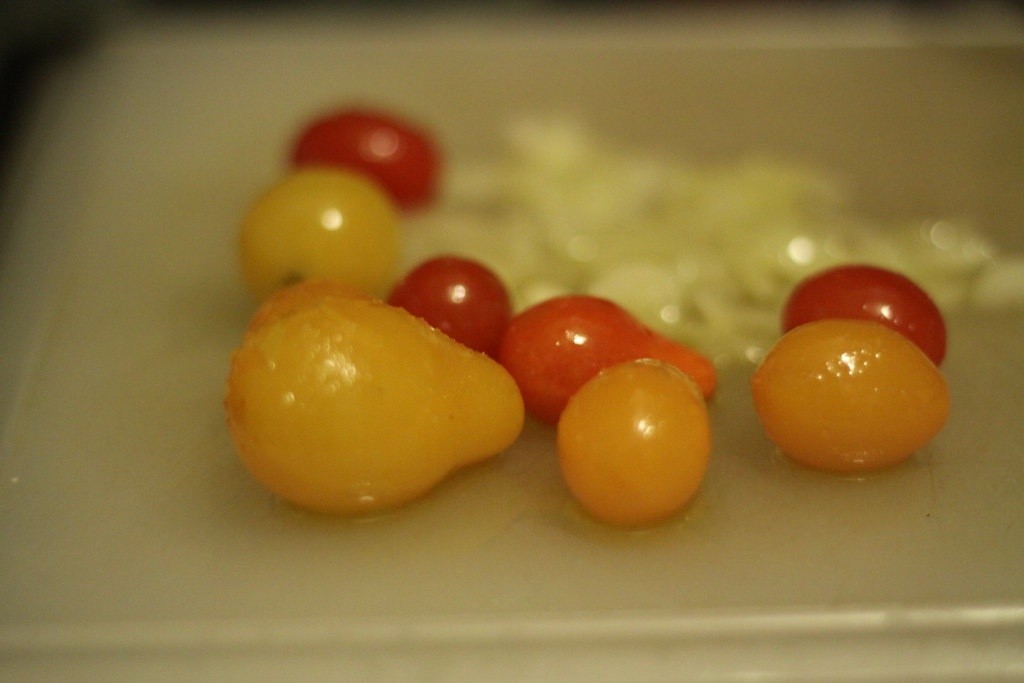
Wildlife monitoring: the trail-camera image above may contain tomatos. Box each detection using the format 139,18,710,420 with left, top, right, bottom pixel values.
223,108,948,526
